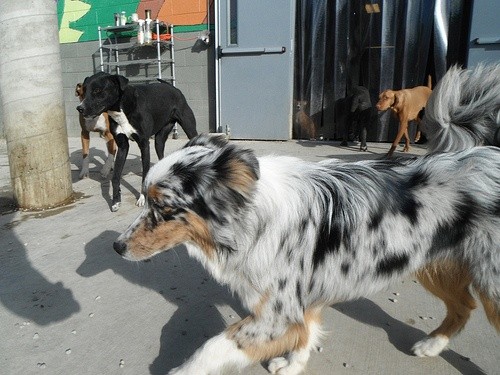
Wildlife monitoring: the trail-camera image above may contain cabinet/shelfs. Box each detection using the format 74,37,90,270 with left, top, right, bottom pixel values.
98,19,179,140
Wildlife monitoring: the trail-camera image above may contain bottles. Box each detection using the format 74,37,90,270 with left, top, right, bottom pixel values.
121,11,125,25
145,19,152,43
115,14,119,26
137,20,145,44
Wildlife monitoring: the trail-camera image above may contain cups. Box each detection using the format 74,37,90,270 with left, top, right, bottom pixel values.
128,13,138,24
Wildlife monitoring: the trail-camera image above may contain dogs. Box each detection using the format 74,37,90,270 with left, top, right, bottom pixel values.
293,75,433,156
113,62,500,375
76,72,199,212
